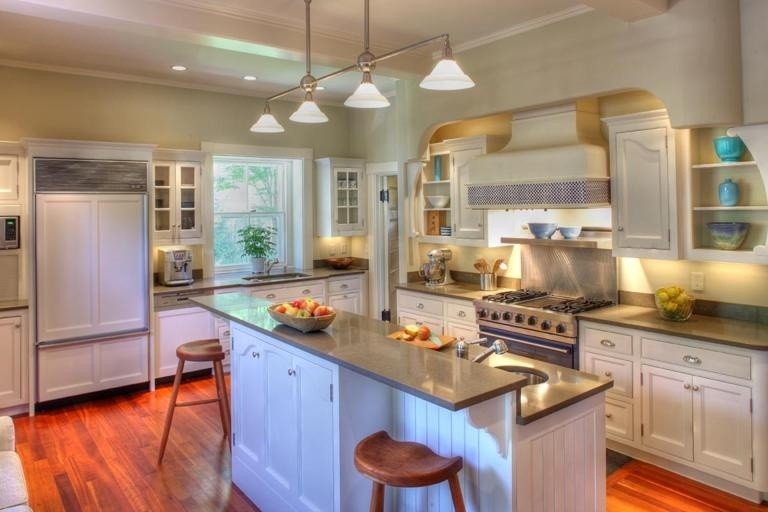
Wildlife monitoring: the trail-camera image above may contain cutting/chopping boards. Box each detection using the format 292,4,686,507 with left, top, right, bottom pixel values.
384,330,457,350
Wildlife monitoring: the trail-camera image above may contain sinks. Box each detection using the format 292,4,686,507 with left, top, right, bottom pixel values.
494,366,548,385
242,273,312,282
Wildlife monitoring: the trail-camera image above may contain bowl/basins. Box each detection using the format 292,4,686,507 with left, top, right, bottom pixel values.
425,196,450,207
655,294,695,321
713,136,746,162
560,226,582,240
707,222,749,250
528,223,558,239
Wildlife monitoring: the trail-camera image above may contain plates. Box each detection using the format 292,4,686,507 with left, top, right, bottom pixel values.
440,227,451,230
440,232,451,236
440,230,451,232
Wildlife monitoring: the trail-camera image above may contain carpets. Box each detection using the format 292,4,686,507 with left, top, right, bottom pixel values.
606,448,632,480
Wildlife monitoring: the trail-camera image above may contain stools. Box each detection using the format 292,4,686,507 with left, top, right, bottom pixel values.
353,430,466,512
156,338,231,465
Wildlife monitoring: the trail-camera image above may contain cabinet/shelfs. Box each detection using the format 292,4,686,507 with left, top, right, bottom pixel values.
454,148,489,248
583,327,634,444
690,123,767,266
328,272,368,318
31,155,148,402
232,328,334,512
153,290,215,379
406,133,451,243
482,213,615,252
447,302,478,341
641,338,751,482
212,287,244,376
153,147,206,247
396,294,443,336
599,107,682,262
314,157,367,238
249,279,328,306
0,141,35,421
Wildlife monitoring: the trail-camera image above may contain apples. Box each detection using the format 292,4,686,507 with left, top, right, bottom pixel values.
405,324,418,337
275,297,328,318
418,325,430,341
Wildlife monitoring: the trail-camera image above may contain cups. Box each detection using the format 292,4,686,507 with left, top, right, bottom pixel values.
418,263,446,286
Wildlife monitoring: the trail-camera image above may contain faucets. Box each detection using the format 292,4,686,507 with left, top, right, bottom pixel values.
456,337,507,363
264,258,279,276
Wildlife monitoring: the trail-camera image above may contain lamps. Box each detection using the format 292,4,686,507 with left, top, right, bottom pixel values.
249,99,285,133
419,33,476,91
288,0,329,123
343,0,391,109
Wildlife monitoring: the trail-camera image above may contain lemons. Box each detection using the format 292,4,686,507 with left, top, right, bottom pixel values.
655,285,689,313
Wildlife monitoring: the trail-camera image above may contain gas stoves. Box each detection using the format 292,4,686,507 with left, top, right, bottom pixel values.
482,288,612,315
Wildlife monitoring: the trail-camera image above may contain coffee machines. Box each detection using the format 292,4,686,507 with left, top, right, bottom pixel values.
157,246,194,286
